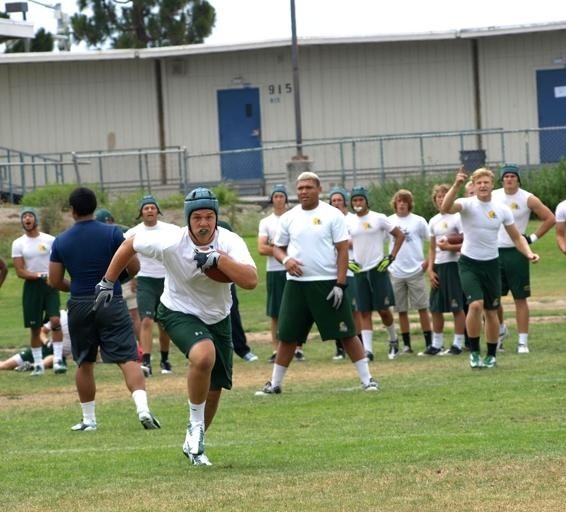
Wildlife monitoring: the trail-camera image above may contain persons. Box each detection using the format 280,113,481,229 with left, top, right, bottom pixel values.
259,185,306,361
0,257,9,290
123,196,181,377
11,208,68,375
92,186,259,467
46,187,161,432
216,220,260,363
94,208,143,365
1,308,73,371
254,171,378,397
328,164,566,369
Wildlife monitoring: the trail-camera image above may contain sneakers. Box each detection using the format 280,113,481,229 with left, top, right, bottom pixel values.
186,417,205,455
361,377,378,392
364,326,529,368
138,411,161,430
52,361,67,374
254,381,282,396
30,365,44,376
182,442,212,467
138,349,345,378
71,418,97,431
13,361,33,371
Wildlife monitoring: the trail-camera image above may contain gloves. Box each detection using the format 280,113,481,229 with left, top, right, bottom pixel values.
522,233,532,245
326,283,346,310
194,245,220,273
376,254,395,273
348,259,360,274
92,276,115,312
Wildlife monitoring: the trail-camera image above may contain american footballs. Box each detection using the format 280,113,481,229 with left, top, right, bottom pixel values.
199,246,236,282
441,233,463,244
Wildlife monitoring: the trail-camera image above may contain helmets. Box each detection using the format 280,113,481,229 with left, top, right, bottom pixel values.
270,184,287,204
92,208,114,223
350,186,368,208
500,162,522,183
329,186,348,208
20,208,38,227
135,196,163,220
184,187,219,231
